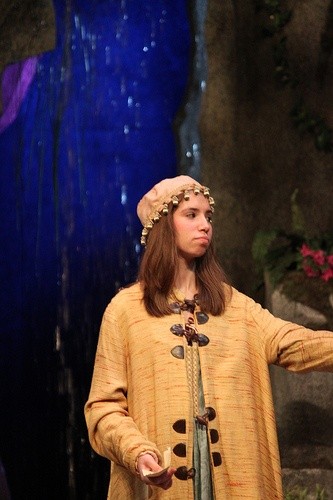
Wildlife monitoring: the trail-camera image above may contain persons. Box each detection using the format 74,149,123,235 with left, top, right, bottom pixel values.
84,175,333,500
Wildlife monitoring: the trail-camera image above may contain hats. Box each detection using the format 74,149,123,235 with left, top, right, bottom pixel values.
136,174,215,246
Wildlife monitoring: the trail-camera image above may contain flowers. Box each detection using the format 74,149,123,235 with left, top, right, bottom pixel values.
277,245,333,309
